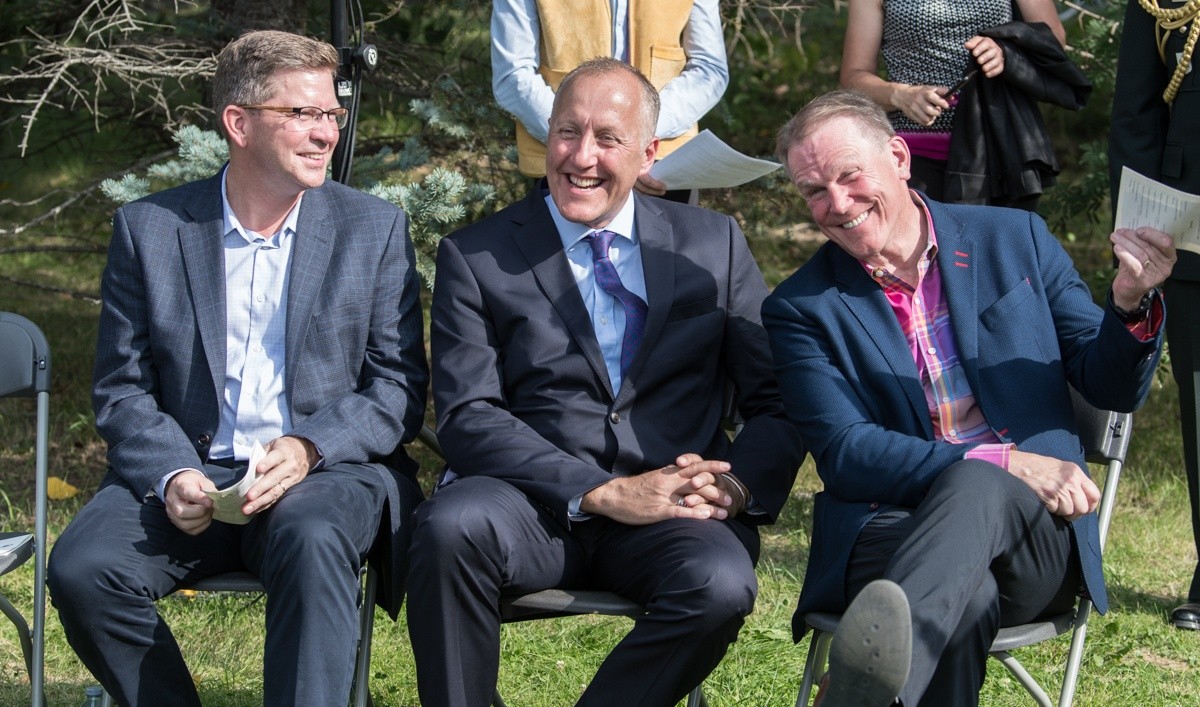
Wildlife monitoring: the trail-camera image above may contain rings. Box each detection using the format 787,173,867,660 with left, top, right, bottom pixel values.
279,481,287,493
1143,259,1151,269
678,496,689,508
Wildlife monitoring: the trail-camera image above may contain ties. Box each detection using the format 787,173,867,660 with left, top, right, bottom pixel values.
582,231,647,387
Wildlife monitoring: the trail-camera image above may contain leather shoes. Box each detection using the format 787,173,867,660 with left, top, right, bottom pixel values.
1170,601,1200,629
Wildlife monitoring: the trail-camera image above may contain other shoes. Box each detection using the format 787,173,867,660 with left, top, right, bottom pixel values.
814,578,912,706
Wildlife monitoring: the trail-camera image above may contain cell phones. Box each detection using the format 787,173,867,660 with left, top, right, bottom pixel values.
941,69,978,100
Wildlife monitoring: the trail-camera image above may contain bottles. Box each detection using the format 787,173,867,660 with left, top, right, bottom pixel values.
83,686,105,707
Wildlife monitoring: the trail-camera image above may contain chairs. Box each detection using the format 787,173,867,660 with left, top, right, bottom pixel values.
793,380,1132,707
182,558,385,706
427,425,709,707
0,312,52,706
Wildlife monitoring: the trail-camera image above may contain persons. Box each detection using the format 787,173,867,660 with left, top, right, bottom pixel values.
840,0,1069,210
45,28,431,707
400,56,810,707
490,0,730,206
1105,0,1199,631
760,90,1179,707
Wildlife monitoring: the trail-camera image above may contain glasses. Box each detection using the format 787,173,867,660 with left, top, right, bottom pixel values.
237,105,349,130
